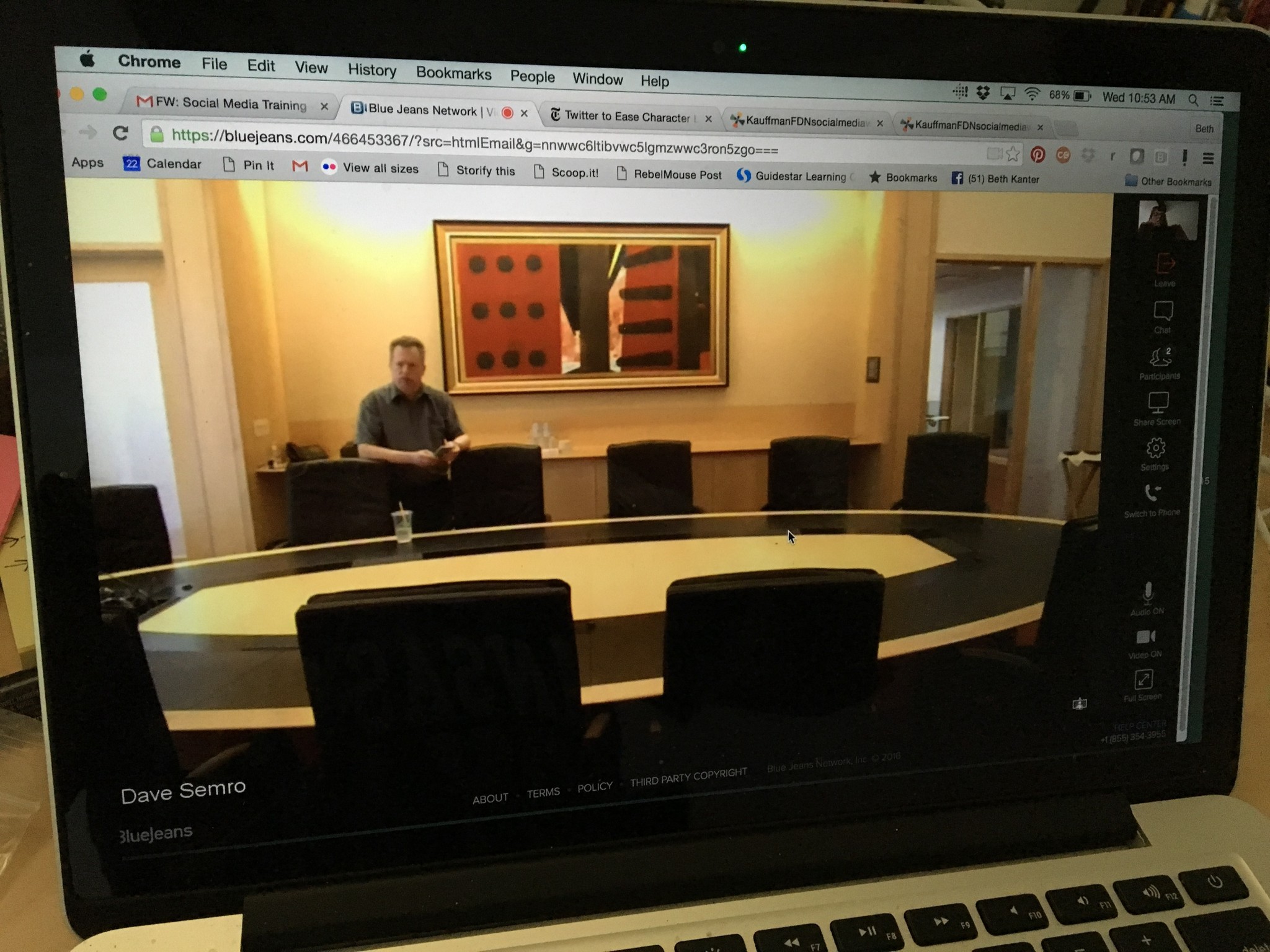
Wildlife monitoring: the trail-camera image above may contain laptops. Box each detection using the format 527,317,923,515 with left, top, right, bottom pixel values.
0,0,1270,952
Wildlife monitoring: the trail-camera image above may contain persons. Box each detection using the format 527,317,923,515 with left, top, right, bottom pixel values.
355,336,471,536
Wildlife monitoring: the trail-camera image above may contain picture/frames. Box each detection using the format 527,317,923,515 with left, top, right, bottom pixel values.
433,219,731,397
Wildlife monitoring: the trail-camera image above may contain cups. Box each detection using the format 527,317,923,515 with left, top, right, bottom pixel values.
392,511,413,543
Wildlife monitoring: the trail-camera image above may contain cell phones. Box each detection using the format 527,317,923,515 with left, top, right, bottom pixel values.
433,444,453,460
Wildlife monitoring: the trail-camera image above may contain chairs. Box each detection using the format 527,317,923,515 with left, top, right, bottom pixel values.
890,432,991,516
450,443,545,528
663,568,885,717
760,434,851,512
602,439,703,518
287,459,394,547
91,484,173,573
294,581,582,733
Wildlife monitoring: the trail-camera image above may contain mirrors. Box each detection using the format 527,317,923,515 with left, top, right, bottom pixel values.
973,308,1020,448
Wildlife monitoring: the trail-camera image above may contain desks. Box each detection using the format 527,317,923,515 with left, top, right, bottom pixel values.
96,508,1069,730
